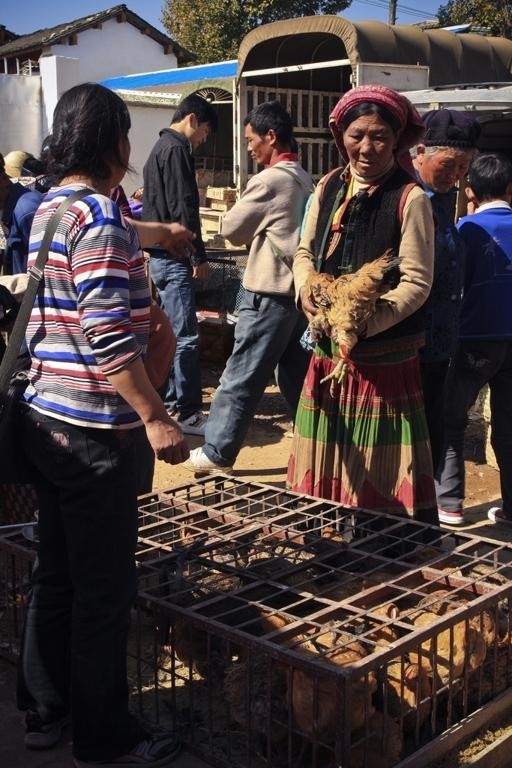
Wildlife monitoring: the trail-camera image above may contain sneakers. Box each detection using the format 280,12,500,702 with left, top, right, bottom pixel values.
437,508,465,524
24,705,181,768
488,507,512,524
177,412,208,435
180,447,234,475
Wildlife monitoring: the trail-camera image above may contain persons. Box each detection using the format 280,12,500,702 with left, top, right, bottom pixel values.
410,109,478,473
16,83,182,767
183,101,314,476
142,93,209,436
1,134,56,607
130,186,144,221
107,183,132,219
287,83,441,529
429,154,511,527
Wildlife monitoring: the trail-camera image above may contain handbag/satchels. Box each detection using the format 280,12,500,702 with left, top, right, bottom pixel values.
0,372,32,483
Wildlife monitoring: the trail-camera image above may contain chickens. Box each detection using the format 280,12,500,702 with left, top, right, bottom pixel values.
304,246,402,399
125,502,512,768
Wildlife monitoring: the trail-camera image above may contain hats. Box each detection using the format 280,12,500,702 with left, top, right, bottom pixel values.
420,109,481,147
